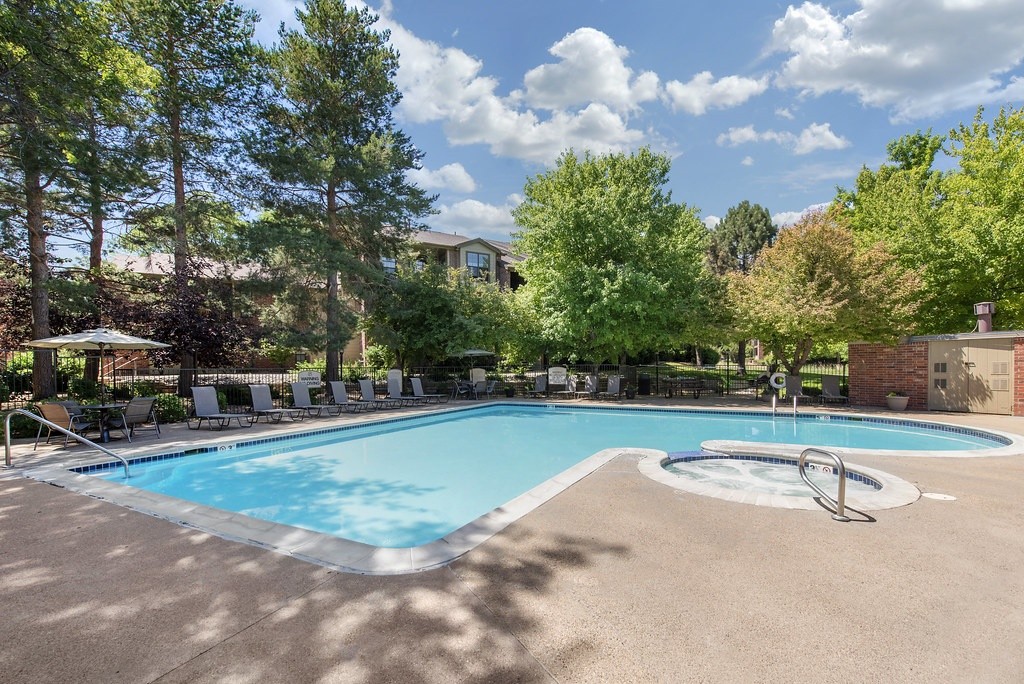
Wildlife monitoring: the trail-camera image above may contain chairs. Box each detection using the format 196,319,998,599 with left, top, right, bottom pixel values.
819,375,848,407
245,384,304,425
33,404,78,449
386,378,423,407
447,375,621,402
110,395,160,443
785,375,812,406
327,381,373,416
187,386,255,431
51,401,94,438
289,382,342,418
410,378,446,405
356,380,396,411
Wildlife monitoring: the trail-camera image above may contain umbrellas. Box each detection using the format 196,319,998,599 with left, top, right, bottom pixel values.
19,327,173,442
447,347,495,400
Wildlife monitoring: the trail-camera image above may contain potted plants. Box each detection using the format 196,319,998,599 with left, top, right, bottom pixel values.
884,390,909,411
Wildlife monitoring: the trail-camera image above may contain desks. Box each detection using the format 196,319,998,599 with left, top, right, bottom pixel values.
76,404,128,442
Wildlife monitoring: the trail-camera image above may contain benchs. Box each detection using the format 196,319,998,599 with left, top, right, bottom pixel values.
649,376,724,398
